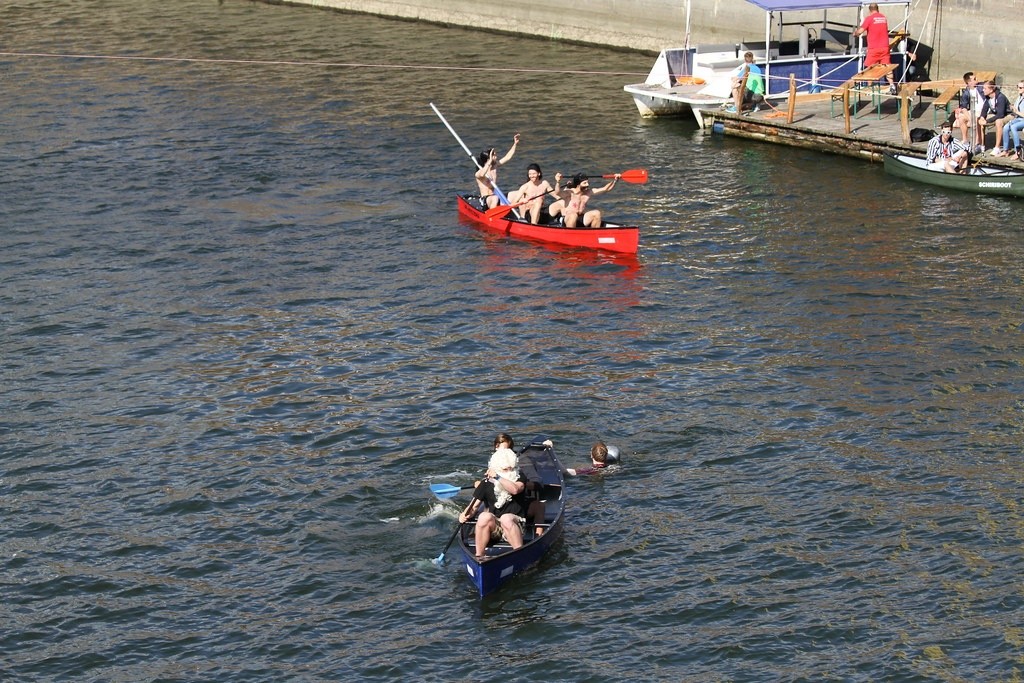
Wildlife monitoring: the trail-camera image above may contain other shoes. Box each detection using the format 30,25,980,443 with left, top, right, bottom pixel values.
889,85,896,95
961,140,969,145
865,87,872,97
995,151,1009,159
1010,152,1020,160
975,143,980,153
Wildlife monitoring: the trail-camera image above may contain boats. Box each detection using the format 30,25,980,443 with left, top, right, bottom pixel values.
624,0,911,128
457,193,639,254
458,435,566,600
883,148,1024,197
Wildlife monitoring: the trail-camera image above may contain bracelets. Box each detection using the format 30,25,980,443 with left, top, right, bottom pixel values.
496,475,501,481
556,182,559,183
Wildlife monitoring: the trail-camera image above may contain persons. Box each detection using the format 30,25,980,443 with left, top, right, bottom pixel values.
926,122,971,174
459,433,545,556
853,3,897,95
955,72,1024,160
475,134,524,218
543,440,608,476
725,51,764,113
511,164,560,225
554,172,621,229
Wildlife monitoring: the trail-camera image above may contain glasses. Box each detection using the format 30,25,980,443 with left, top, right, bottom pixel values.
1016,85,1024,90
942,130,952,135
970,76,976,80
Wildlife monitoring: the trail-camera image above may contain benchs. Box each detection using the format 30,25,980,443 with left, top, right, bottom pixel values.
897,82,921,120
932,87,960,127
831,79,860,118
473,499,559,527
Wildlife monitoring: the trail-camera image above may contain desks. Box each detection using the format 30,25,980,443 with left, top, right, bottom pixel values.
975,72,996,84
851,63,899,119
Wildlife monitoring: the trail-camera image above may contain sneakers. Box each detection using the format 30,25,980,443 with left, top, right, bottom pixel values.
726,106,743,113
981,146,985,151
751,101,760,112
990,147,1001,156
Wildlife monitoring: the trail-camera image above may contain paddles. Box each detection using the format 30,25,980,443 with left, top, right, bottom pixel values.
485,183,570,222
560,169,648,184
436,473,490,564
429,483,476,499
